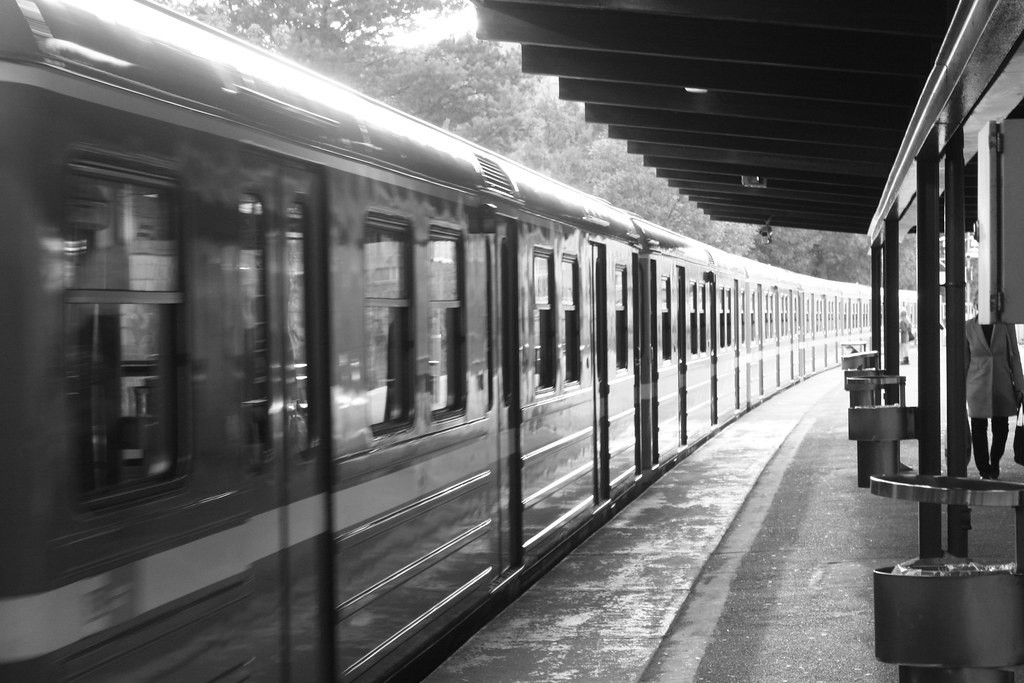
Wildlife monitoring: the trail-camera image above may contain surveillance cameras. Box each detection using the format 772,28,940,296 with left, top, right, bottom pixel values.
761,226,772,238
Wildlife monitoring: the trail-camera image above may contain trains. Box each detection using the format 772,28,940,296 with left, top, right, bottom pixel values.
0,0,974,683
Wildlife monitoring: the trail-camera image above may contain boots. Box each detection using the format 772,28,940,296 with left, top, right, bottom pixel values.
900,357,909,365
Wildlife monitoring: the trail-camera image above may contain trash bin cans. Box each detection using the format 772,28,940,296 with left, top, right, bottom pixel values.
847,376,905,489
842,351,881,407
870,473,1024,683
841,342,866,353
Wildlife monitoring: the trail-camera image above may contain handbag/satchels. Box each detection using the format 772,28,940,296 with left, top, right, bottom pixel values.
907,328,914,341
1013,392,1024,466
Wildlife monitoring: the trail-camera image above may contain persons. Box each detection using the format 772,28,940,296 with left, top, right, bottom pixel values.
965,286,1024,478
899,310,912,365
